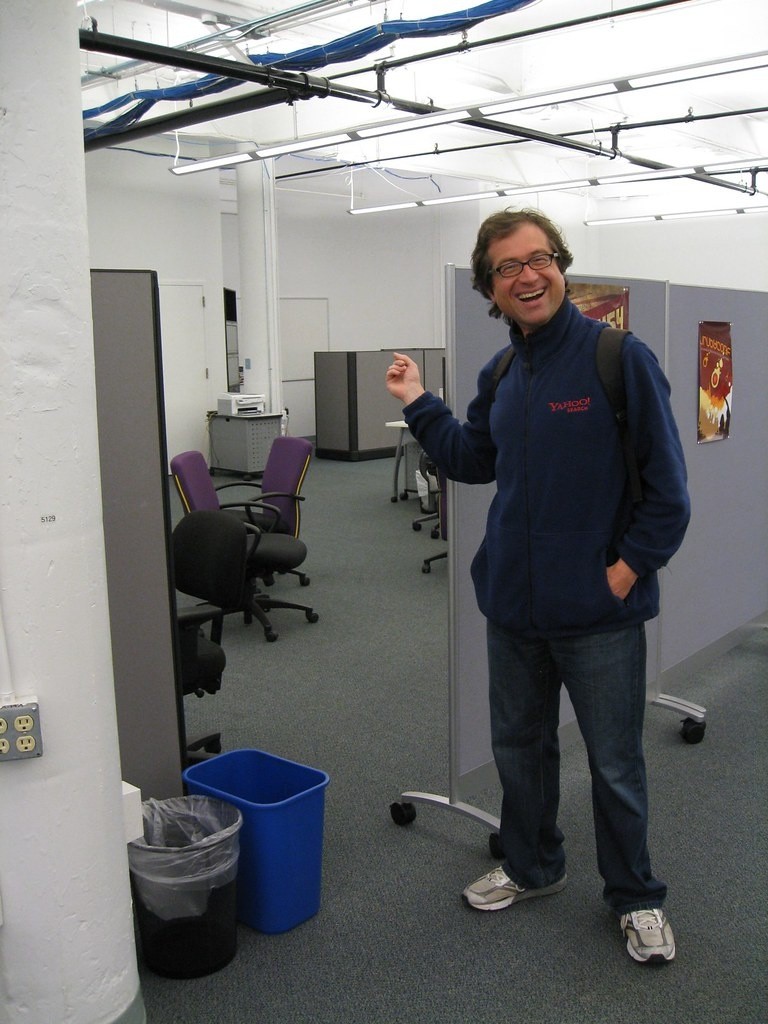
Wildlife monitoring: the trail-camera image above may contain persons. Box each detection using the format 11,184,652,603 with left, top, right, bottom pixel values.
386,206,690,964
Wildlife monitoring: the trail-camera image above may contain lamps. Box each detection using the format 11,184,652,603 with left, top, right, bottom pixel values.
344,155,768,215
169,49,768,175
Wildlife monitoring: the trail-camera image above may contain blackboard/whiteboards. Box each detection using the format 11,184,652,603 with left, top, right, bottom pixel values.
276,297,331,382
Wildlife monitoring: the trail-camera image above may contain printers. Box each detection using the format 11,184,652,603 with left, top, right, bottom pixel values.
217,392,266,416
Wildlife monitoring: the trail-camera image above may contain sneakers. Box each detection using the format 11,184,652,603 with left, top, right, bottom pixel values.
462,866,567,910
608,906,675,964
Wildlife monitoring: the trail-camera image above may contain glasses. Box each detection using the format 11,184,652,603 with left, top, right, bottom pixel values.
489,252,559,278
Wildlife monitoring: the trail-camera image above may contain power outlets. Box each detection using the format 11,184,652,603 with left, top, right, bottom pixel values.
0,696,43,762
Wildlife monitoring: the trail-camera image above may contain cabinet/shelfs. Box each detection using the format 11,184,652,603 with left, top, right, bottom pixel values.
387,420,418,504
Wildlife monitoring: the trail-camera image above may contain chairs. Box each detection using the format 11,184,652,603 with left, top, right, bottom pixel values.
250,434,315,538
172,510,247,698
410,452,447,575
170,450,318,642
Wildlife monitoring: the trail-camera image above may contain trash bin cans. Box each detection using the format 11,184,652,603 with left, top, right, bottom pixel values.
181,748,331,935
127,793,243,980
